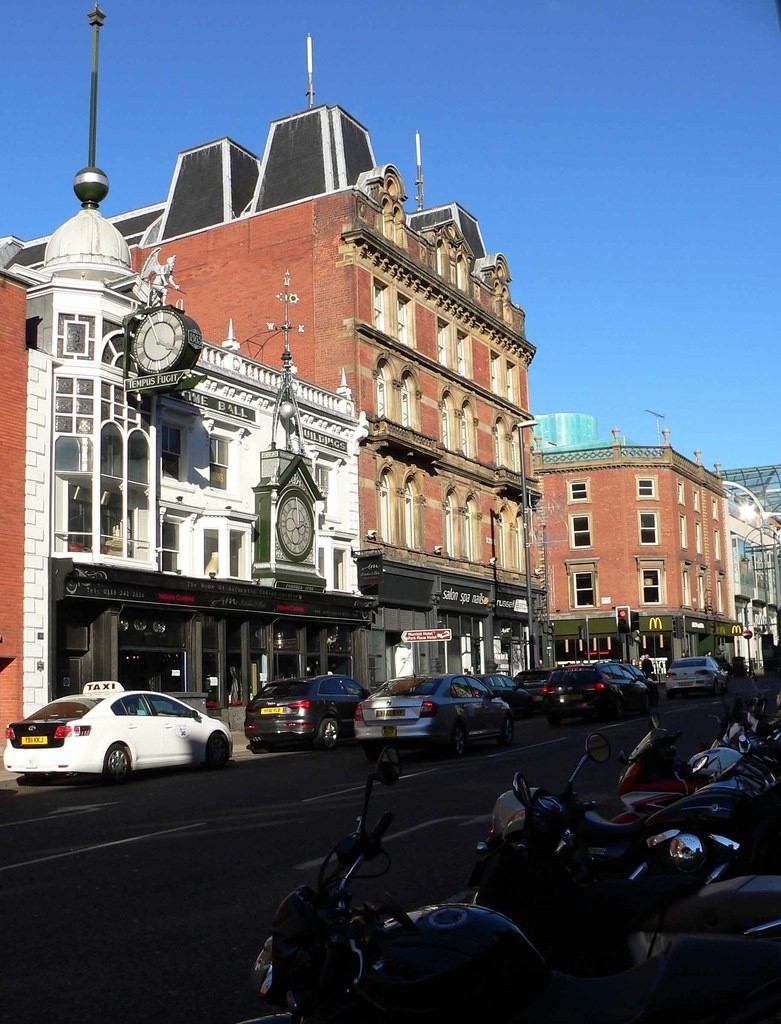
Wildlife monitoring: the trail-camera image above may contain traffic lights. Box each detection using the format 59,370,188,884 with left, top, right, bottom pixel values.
617,609,629,633
672,615,682,639
630,611,640,632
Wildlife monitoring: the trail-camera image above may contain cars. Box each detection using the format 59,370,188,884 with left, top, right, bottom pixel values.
5,680,232,787
354,674,514,764
474,672,535,718
514,666,558,710
665,656,729,701
622,664,658,707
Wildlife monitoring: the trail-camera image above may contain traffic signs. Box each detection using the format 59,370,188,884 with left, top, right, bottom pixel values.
400,628,453,644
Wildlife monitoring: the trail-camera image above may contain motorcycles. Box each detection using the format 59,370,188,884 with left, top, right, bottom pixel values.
236,675,781,1024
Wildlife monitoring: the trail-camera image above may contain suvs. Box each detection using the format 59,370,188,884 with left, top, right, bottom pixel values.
540,659,652,729
244,674,372,755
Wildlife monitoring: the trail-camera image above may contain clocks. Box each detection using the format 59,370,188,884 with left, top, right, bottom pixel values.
277,488,314,563
133,309,185,374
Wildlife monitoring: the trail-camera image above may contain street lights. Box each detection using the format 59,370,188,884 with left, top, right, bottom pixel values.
516,419,540,671
740,526,781,658
